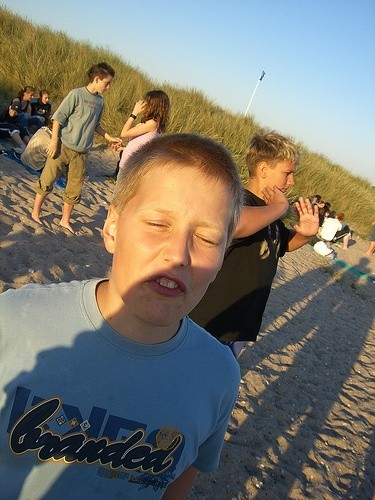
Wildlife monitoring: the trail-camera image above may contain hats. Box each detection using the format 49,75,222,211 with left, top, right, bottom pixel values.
11,104,20,112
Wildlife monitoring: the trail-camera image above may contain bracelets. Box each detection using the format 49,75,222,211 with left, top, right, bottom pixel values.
130,114,137,120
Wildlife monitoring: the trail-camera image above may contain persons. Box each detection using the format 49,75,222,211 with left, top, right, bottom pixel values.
0,85,51,149
20,116,90,182
308,194,350,250
189,127,320,361
111,90,170,181
29,62,123,234
365,221,375,256
0,132,241,499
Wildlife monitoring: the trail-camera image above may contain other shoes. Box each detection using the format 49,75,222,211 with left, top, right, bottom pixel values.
103,175,117,185
